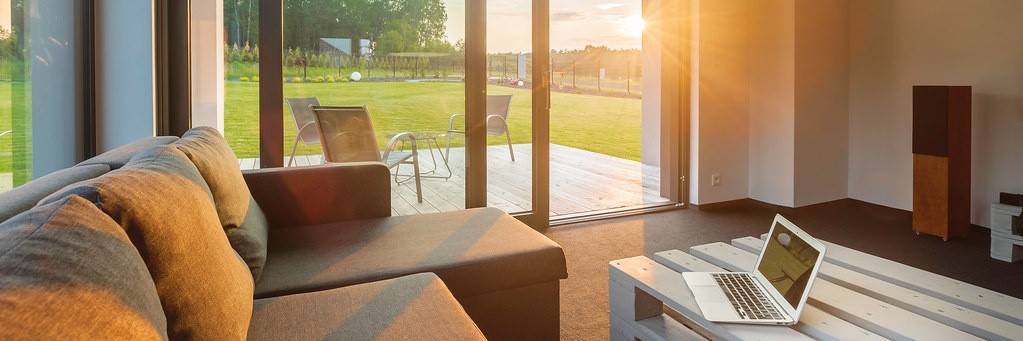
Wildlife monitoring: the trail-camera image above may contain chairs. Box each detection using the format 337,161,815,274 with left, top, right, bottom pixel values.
282,96,325,169
443,93,514,165
307,104,422,204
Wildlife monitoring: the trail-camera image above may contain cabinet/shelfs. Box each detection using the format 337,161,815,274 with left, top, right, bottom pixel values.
988,202,1022,264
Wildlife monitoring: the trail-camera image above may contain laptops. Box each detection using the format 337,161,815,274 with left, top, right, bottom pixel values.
682,213,828,326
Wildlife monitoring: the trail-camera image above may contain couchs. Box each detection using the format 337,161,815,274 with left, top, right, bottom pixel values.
0,126,571,341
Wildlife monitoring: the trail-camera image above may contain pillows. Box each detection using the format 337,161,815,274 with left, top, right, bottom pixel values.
32,145,256,341
0,191,170,341
165,126,269,285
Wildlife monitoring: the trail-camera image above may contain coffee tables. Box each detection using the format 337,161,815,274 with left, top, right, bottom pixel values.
608,233,1023,341
384,131,452,185
